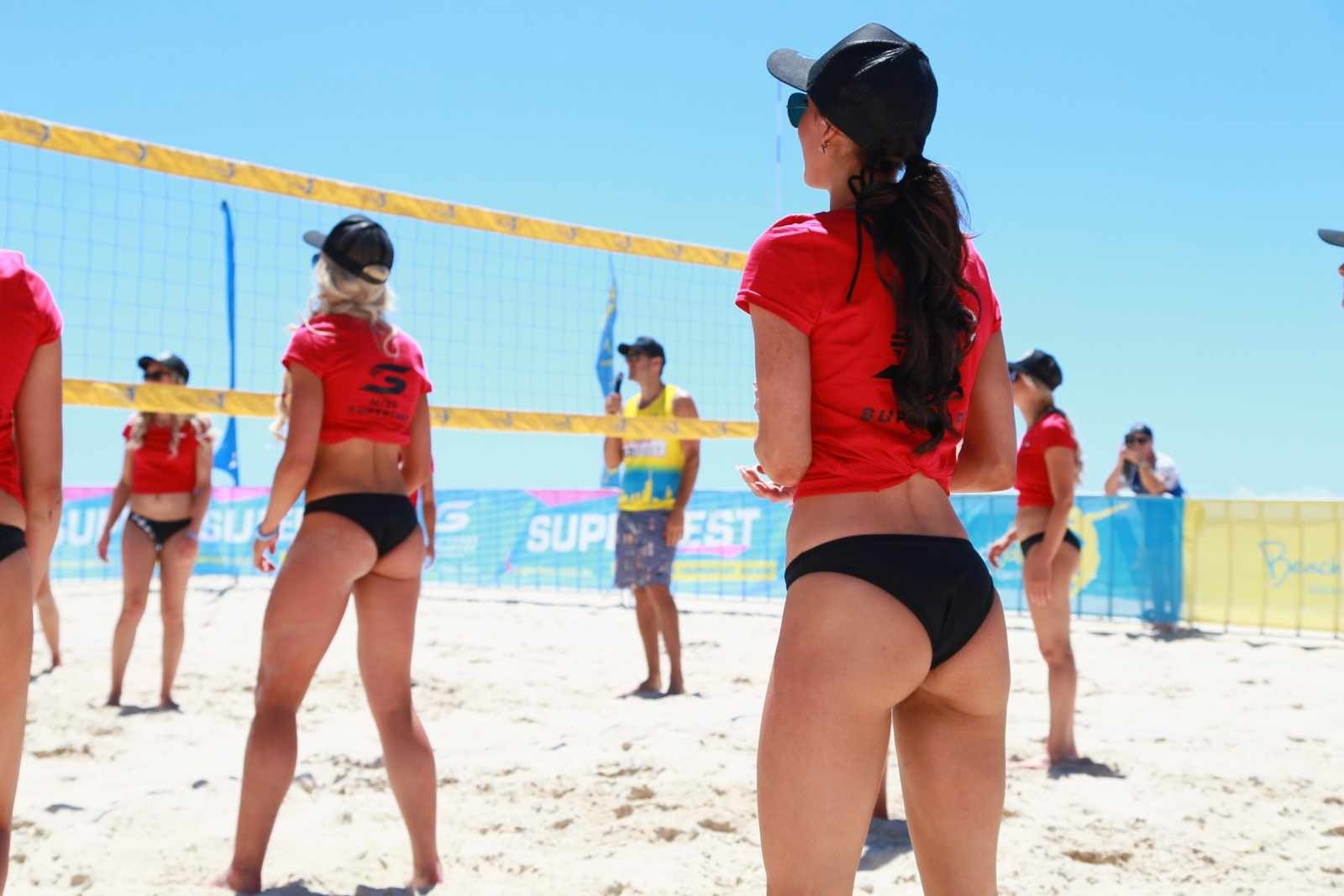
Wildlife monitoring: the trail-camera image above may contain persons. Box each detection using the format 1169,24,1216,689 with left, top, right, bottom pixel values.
989,349,1083,767
0,249,63,896
603,336,700,697
733,20,1019,895
209,212,442,895
1103,425,1184,632
1317,228,1344,278
96,353,213,713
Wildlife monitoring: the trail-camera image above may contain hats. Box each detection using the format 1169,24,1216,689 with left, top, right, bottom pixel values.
767,22,938,162
1007,349,1063,390
1129,424,1152,439
138,352,189,381
303,215,394,284
618,337,664,359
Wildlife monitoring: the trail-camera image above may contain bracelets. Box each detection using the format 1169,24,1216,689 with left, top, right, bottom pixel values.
183,531,199,542
253,523,279,542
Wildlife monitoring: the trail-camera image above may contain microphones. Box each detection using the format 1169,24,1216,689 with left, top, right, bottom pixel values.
615,373,623,394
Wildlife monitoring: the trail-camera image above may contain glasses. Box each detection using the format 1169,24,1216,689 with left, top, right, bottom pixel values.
624,353,640,364
312,254,320,267
143,370,171,382
1135,437,1147,444
785,92,816,128
1010,370,1023,382
